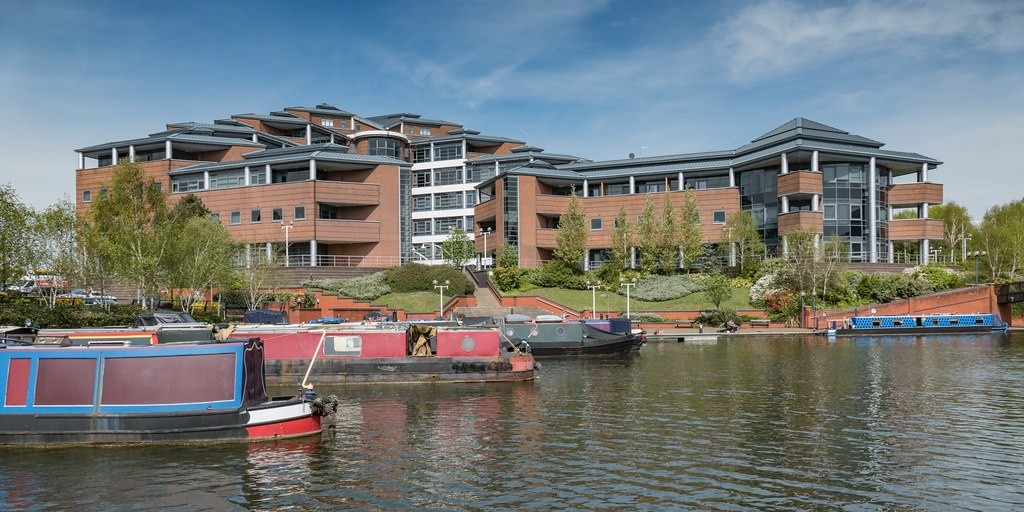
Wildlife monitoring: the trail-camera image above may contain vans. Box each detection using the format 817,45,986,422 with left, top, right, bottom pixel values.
7,274,68,297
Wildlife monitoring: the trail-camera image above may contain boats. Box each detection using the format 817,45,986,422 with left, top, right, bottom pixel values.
0,308,647,386
0,335,338,448
834,311,1010,338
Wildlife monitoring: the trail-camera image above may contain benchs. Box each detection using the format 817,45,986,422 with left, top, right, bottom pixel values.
674,320,695,328
750,319,770,328
631,320,642,329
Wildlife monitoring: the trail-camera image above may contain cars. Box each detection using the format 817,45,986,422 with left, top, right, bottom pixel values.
57,288,119,307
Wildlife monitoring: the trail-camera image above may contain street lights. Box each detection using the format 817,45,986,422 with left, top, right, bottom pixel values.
279,220,293,268
432,279,450,319
812,288,818,331
801,290,806,328
967,250,987,284
479,226,492,271
585,279,602,319
620,276,637,319
